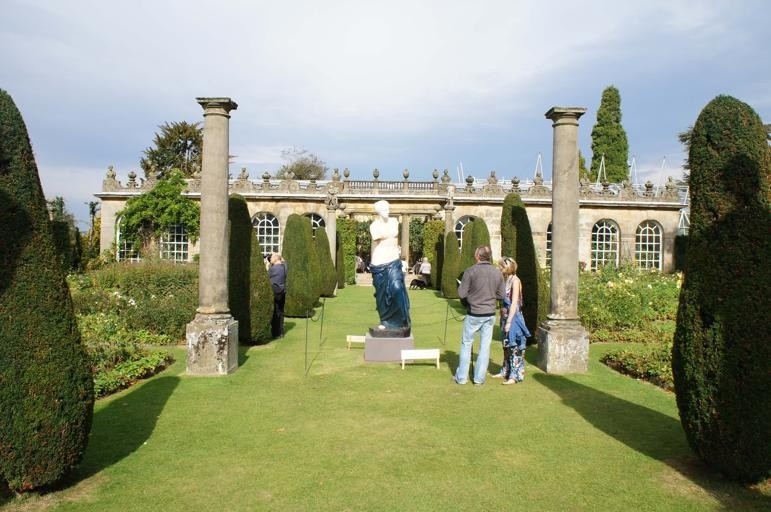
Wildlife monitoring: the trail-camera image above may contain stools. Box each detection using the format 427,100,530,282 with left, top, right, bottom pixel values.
400,348,442,371
346,334,365,350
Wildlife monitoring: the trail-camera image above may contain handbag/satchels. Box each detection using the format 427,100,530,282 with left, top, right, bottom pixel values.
273,287,283,301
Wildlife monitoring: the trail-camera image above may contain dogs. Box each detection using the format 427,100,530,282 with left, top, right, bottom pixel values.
409,279,426,290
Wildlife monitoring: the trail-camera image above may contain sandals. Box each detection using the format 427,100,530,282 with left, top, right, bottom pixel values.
491,373,504,378
502,378,516,385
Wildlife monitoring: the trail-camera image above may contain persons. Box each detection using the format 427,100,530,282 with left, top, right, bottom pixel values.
665,176,677,191
419,257,432,286
106,164,116,180
192,166,202,179
284,164,295,180
456,246,506,386
146,165,160,180
332,168,341,181
487,170,498,184
580,172,590,186
370,200,410,330
239,166,249,180
492,258,527,385
441,168,451,183
623,175,634,190
533,170,544,185
268,252,287,339
